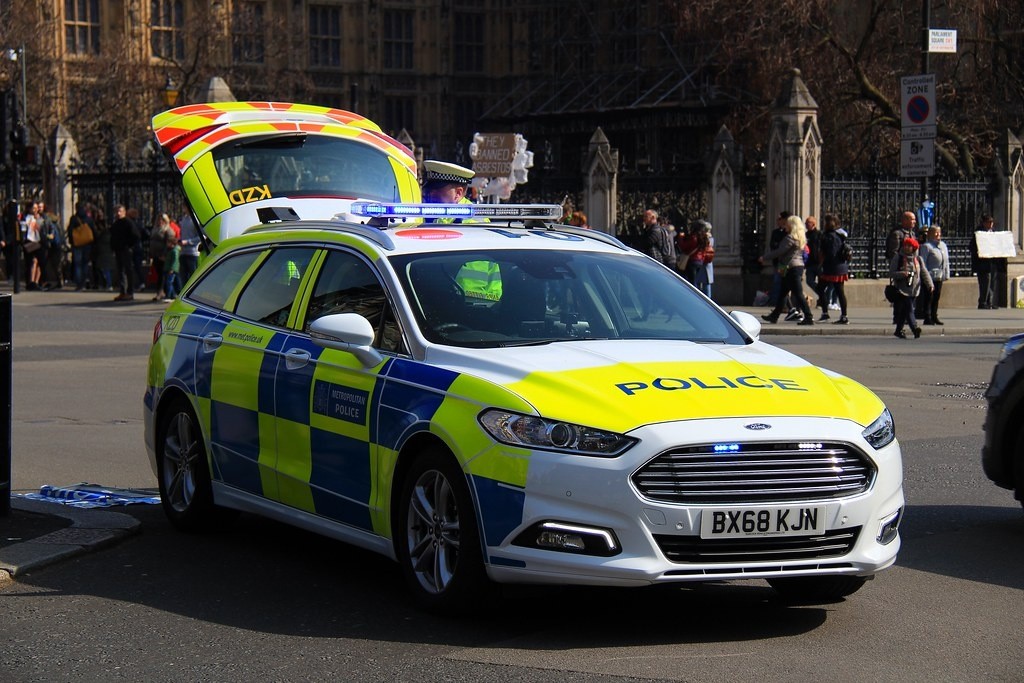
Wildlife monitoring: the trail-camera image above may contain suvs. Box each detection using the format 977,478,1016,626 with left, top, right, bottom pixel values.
144,101,905,622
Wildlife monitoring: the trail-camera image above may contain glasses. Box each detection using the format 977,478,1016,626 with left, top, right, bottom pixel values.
424,180,447,190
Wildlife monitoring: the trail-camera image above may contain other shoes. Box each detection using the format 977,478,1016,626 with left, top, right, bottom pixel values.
761,311,779,324
893,328,908,340
911,327,922,339
978,301,999,309
815,312,831,323
796,316,812,325
924,316,944,326
833,315,848,325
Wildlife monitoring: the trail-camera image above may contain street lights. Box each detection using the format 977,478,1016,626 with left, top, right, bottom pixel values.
6,45,23,198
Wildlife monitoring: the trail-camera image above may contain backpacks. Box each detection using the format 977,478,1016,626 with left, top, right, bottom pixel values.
704,246,714,265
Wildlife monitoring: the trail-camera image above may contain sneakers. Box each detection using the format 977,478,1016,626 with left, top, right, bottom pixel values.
784,307,803,321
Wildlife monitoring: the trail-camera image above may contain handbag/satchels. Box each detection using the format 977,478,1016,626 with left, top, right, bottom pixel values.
676,252,690,271
884,284,900,303
72,216,94,247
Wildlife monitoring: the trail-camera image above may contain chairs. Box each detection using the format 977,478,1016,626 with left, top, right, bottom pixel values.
495,265,566,338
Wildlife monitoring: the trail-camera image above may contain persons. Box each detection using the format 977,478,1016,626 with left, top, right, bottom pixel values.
563,194,1008,340
420,160,511,334
0,192,207,305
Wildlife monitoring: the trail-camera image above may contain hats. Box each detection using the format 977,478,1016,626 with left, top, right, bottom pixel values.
903,237,919,248
423,159,474,182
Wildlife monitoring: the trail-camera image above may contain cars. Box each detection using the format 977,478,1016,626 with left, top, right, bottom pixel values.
981,333,1024,508
